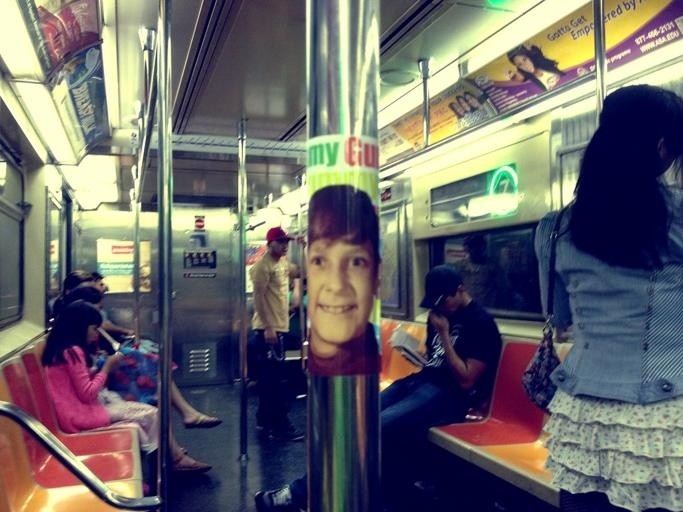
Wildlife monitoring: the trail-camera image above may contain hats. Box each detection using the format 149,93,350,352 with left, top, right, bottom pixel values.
267,227,295,243
420,264,463,308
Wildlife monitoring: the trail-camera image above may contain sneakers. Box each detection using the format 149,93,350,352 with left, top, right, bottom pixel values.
256,417,269,429
256,485,293,511
272,426,304,441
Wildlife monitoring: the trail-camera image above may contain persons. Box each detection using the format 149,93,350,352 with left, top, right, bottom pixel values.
448,44,566,130
307,184,381,359
254,264,502,512
40,269,224,473
248,225,307,442
534,84,683,512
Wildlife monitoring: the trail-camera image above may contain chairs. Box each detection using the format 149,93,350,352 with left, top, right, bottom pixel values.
0,331,164,512
376,316,575,508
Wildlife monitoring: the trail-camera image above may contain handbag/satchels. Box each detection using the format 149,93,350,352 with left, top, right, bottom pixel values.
522,331,562,416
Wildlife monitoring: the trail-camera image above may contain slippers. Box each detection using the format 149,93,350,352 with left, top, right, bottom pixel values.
185,414,224,428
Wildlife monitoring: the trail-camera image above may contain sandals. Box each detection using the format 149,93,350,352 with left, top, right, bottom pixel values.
174,448,212,474
